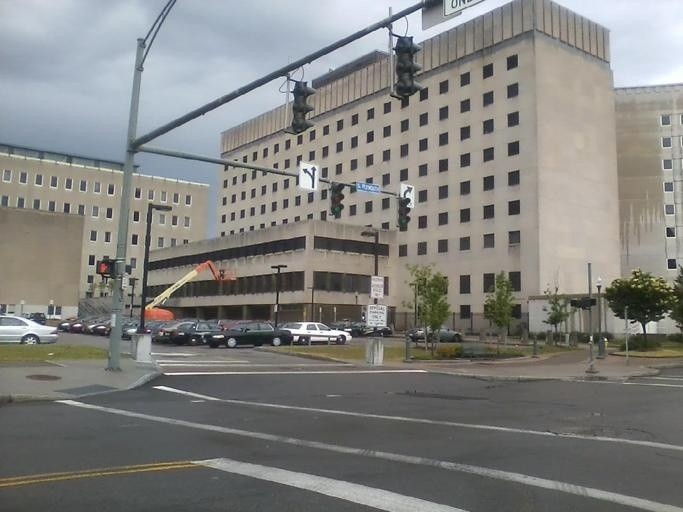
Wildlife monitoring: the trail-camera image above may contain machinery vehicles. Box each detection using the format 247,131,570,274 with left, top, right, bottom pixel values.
143,258,238,320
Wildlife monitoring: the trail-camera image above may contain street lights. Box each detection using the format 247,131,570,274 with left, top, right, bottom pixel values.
270,264,288,328
136,202,172,334
585,258,606,374
360,229,380,338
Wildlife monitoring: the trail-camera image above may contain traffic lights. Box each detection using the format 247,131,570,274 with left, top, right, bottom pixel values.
399,197,411,232
332,181,345,219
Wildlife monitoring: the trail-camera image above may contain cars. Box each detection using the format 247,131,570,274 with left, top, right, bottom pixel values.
0,315,60,345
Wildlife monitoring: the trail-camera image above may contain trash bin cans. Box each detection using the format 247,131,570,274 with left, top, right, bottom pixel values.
599,337,608,356
366,337,384,366
545,330,553,345
570,332,578,346
